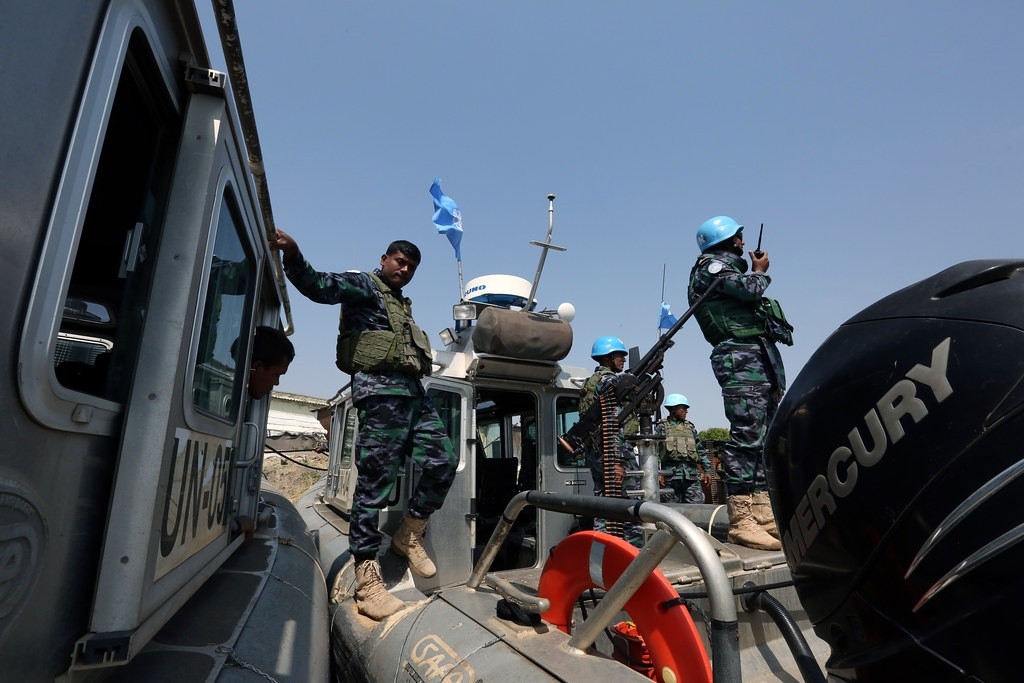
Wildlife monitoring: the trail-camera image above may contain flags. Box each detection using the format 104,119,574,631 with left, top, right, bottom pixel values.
658,299,683,330
428,176,463,261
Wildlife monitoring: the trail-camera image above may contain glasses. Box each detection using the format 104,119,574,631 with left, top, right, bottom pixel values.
734,233,742,242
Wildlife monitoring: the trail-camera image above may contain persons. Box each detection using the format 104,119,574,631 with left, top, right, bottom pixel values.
194,253,252,411
577,335,712,547
270,227,458,622
688,215,789,552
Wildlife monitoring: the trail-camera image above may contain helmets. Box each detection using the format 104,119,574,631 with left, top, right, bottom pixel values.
696,216,744,254
664,394,689,410
591,336,628,362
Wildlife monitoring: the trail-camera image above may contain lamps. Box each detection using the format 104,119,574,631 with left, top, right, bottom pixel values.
438,327,462,346
453,303,478,321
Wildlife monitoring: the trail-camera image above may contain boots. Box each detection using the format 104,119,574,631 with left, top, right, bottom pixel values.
391,514,436,578
751,491,780,540
726,493,781,551
354,560,406,621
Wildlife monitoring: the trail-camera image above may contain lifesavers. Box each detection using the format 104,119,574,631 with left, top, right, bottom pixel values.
536,530,713,683
